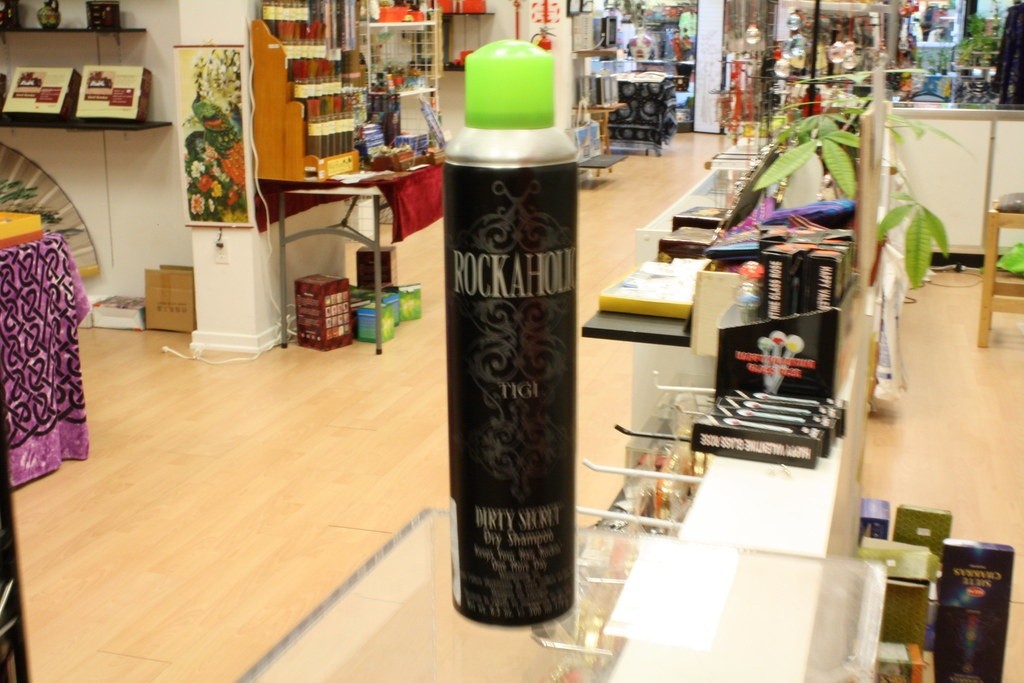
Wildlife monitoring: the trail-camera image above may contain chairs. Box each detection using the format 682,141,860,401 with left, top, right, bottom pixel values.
977,192,1024,349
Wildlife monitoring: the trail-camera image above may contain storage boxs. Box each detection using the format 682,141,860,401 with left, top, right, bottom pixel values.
350,296,371,338
357,302,395,344
361,291,400,325
295,274,353,353
585,123,602,157
2,66,81,120
384,283,422,321
361,282,392,291
356,245,398,286
75,64,152,121
349,284,372,296
143,265,197,332
858,497,1014,683
86,1,121,29
597,204,863,465
565,126,592,163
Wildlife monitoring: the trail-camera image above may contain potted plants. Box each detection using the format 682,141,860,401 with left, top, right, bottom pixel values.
926,53,937,75
938,49,949,75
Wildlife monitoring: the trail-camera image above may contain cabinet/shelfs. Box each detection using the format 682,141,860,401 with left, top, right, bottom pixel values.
411,12,495,71
347,0,439,150
252,18,361,184
952,37,1002,82
888,100,1024,269
0,27,172,132
916,41,958,79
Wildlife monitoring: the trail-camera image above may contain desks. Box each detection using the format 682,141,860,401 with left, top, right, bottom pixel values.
573,103,627,178
591,77,678,155
277,163,444,354
